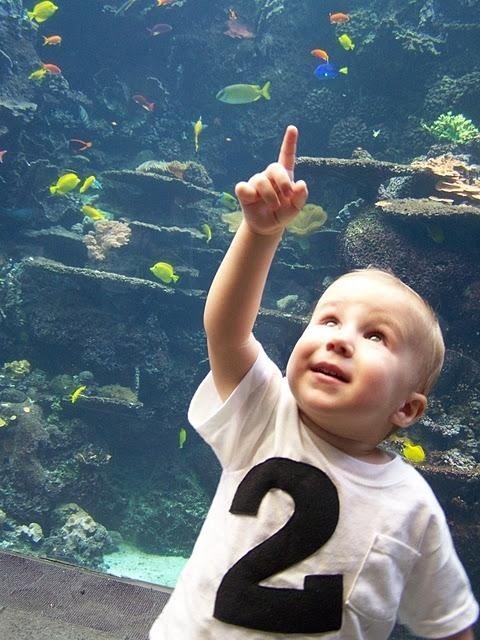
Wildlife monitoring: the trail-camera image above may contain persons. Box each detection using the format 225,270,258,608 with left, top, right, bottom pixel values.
147,122,480,640
